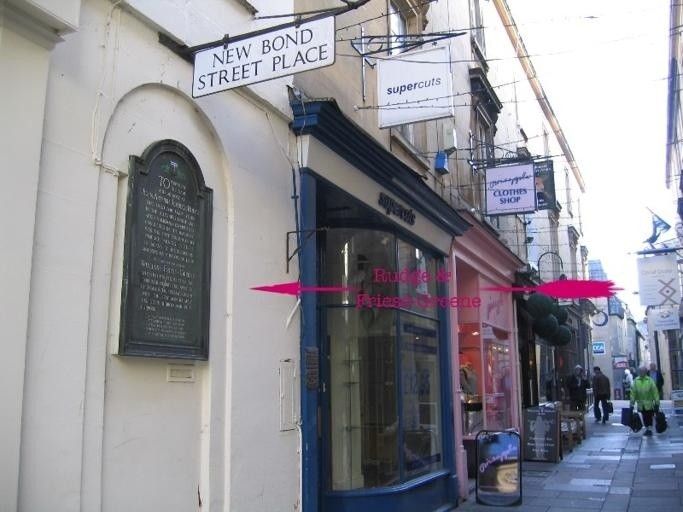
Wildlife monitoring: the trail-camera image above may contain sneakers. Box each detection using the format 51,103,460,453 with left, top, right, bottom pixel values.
643,430,652,437
595,419,606,425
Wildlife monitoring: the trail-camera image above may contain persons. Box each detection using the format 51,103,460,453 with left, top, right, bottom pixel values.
591,365,610,425
544,368,562,401
629,366,660,437
621,362,664,400
565,364,590,420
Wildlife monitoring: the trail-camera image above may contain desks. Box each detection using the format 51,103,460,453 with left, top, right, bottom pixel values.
564,409,587,440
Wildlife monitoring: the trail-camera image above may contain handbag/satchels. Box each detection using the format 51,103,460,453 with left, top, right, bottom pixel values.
607,401,613,412
621,408,642,432
655,411,667,432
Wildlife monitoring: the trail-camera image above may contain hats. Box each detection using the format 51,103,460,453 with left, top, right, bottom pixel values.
575,364,582,369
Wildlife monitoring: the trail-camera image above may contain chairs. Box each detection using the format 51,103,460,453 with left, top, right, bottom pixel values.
555,400,581,452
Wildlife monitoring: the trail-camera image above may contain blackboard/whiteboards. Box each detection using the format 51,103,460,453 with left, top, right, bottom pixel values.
671,389,682,409
118,139,213,361
522,407,560,463
